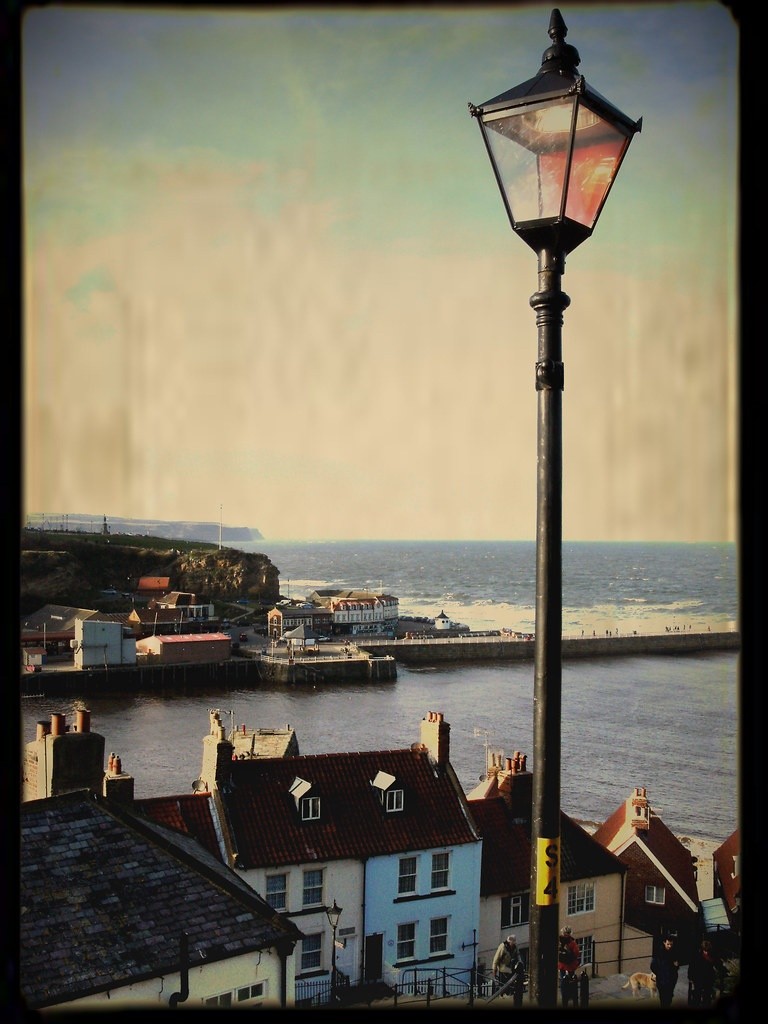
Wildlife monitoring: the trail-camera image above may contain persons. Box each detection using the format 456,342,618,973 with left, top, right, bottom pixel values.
558,926,579,1001
688,936,728,1007
650,936,681,1008
492,935,523,999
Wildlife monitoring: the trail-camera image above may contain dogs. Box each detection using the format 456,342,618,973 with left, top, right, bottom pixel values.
621,973,657,998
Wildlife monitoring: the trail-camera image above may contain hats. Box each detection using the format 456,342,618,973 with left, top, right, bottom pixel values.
507,935,516,944
561,926,571,933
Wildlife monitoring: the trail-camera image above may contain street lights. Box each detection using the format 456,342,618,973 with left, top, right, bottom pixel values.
467,2,643,1005
327,899,343,1005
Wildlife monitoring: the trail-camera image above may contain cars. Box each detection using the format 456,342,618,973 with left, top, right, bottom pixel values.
318,636,331,641
102,589,116,594
239,633,248,641
317,605,325,609
237,620,252,627
237,599,273,605
296,602,316,609
222,621,232,629
26,664,42,672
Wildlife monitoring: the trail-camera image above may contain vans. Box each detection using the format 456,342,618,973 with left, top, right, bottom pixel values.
279,631,292,643
276,600,292,606
